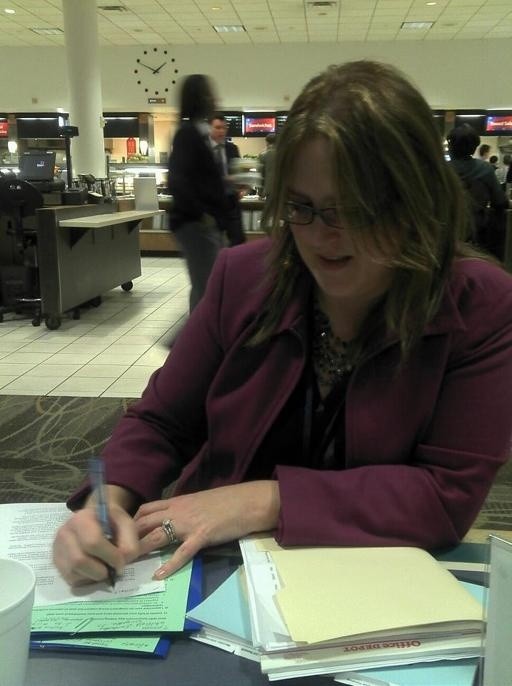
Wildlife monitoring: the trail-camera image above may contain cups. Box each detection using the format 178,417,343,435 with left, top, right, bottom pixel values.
0,556,38,678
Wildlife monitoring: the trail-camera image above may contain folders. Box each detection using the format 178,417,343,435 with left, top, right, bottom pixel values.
29,543,202,661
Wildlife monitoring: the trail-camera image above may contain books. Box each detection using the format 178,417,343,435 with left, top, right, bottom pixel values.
29,634,173,660
234,530,489,682
185,565,489,686
24,542,201,635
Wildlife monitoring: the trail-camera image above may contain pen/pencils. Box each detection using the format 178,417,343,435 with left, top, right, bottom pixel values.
87,458,116,592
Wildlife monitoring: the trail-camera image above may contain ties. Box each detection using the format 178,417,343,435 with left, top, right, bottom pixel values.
218,145,225,177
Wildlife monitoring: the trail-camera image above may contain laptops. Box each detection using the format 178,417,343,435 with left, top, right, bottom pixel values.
19,154,57,182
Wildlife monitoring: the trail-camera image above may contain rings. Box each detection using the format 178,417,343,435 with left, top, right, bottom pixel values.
163,519,176,544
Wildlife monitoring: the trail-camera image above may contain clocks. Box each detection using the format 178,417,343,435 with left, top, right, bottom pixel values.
134,47,178,104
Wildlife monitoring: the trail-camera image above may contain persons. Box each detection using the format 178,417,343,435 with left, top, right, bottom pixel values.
208,112,247,244
495,156,510,191
256,133,279,234
53,61,512,588
165,72,232,350
444,122,512,262
485,153,499,171
478,144,490,161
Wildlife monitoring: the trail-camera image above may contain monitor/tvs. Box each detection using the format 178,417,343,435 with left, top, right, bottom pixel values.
275,114,287,129
486,110,511,136
433,112,445,133
456,114,487,132
226,114,244,135
243,110,277,139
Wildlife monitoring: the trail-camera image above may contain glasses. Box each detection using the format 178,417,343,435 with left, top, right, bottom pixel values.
280,197,375,231
